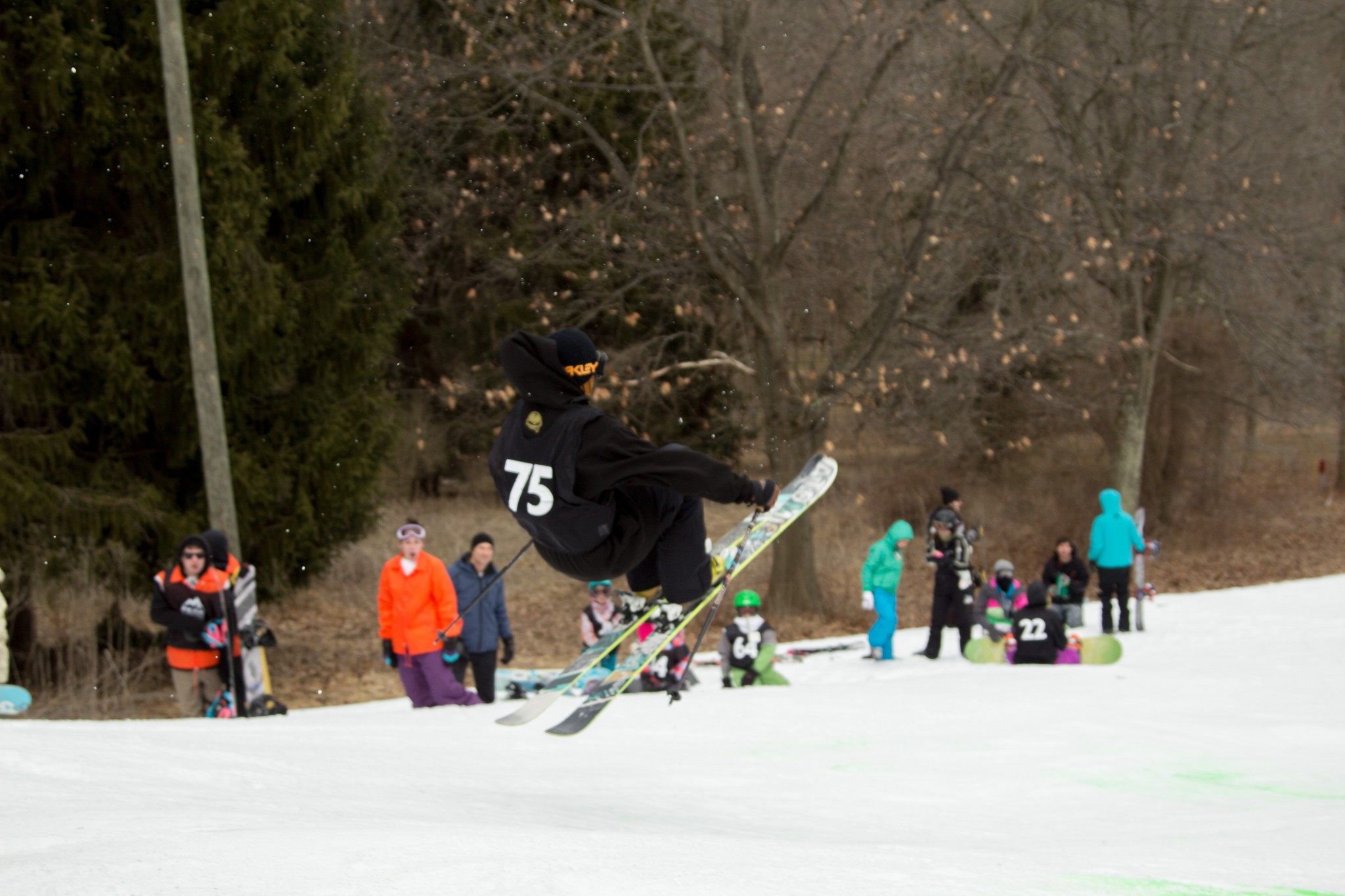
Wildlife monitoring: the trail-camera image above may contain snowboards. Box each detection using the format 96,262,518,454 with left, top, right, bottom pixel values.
226,562,290,717
962,633,1123,665
1133,506,1155,633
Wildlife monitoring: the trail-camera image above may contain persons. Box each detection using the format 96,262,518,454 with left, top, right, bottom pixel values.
1006,582,1071,664
918,517,981,659
1087,487,1149,634
373,517,483,710
487,326,778,602
973,559,1030,641
437,534,518,704
148,534,229,718
1041,536,1092,628
861,517,917,659
194,529,268,717
715,590,788,687
924,485,978,565
576,579,625,656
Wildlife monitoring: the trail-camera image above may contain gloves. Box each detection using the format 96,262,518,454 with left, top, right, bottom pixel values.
861,591,874,611
641,670,757,693
750,478,781,512
441,636,467,666
500,636,515,665
201,616,230,650
455,636,470,662
382,639,397,668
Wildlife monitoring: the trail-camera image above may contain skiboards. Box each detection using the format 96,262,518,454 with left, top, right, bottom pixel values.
495,451,839,738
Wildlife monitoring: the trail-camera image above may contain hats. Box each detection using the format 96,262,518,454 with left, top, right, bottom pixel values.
994,558,1015,572
941,487,960,506
545,327,601,388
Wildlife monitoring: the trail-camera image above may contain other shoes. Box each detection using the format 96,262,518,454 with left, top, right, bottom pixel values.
635,582,664,607
669,554,727,616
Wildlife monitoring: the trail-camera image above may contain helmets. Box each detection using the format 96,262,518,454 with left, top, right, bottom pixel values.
734,588,762,607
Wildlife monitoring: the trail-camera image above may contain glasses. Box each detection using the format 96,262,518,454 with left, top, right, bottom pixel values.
396,524,426,539
183,552,205,559
590,588,610,597
